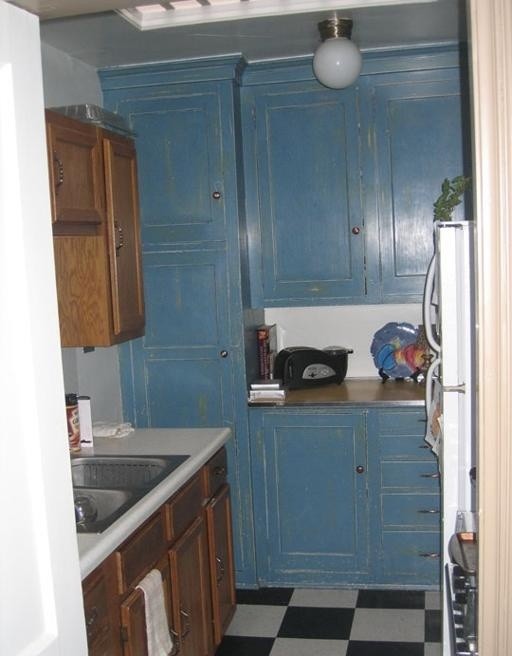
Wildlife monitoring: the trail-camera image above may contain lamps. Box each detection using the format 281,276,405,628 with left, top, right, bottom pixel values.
311,12,363,91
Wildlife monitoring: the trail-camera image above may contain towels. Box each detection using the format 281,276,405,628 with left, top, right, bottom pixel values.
135,567,174,656
93,421,134,439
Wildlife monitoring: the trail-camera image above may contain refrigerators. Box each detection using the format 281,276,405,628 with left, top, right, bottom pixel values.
423,218,476,656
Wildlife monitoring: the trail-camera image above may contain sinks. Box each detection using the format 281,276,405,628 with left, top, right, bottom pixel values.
73,488,141,535
70,453,190,492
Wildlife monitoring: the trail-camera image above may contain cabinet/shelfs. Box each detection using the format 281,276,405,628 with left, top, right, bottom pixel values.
366,408,441,584
46,108,146,347
249,407,371,590
82,473,215,656
95,51,259,590
203,447,238,646
240,39,473,310
